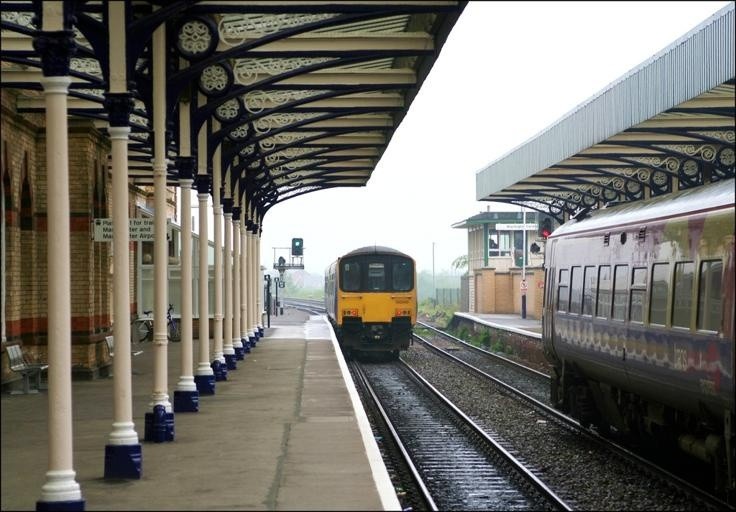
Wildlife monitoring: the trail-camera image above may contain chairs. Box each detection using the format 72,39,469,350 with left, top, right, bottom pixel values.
105,335,145,376
4,341,48,395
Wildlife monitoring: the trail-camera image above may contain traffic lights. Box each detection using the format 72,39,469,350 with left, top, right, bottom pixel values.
292,238,303,256
539,218,552,238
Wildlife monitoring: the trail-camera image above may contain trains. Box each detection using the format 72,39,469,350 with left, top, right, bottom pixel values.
543,178,734,506
324,245,418,362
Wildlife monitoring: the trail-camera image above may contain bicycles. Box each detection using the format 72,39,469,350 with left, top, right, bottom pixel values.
130,304,182,343
